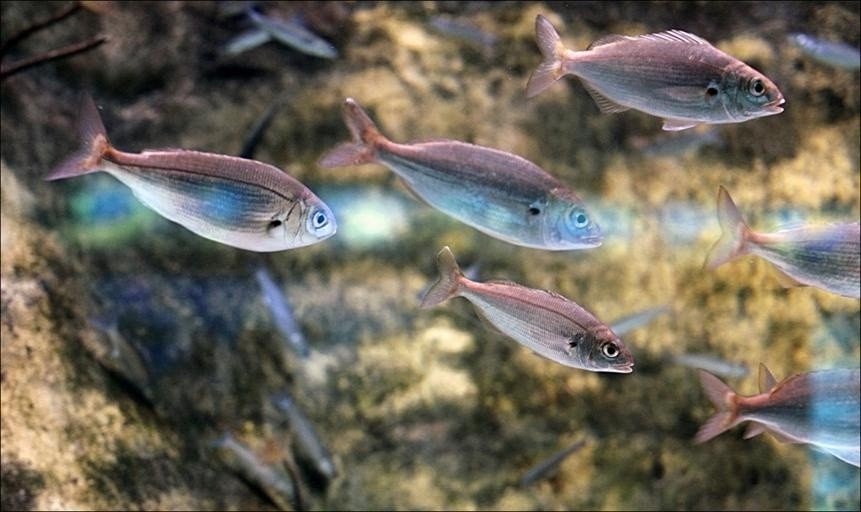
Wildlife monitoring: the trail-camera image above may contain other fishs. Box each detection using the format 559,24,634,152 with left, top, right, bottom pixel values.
220,0,860,509
